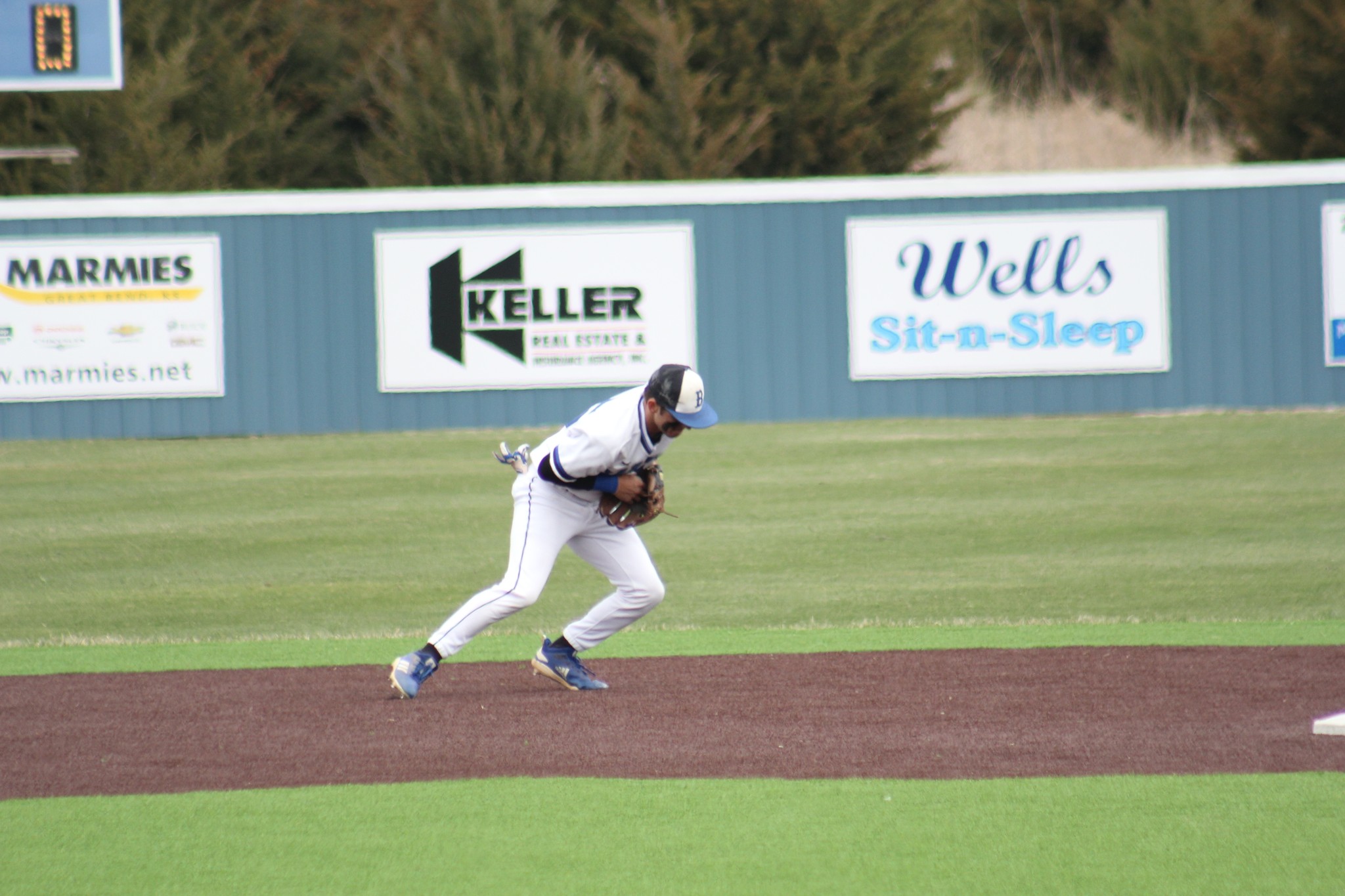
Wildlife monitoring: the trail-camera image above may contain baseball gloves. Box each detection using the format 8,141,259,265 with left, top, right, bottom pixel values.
598,462,665,532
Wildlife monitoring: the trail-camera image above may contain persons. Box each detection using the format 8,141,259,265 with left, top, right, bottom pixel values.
389,363,718,700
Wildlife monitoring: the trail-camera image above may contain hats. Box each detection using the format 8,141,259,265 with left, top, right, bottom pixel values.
648,364,718,429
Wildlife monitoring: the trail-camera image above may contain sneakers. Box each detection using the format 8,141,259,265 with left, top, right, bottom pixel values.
387,653,437,700
532,639,610,689
